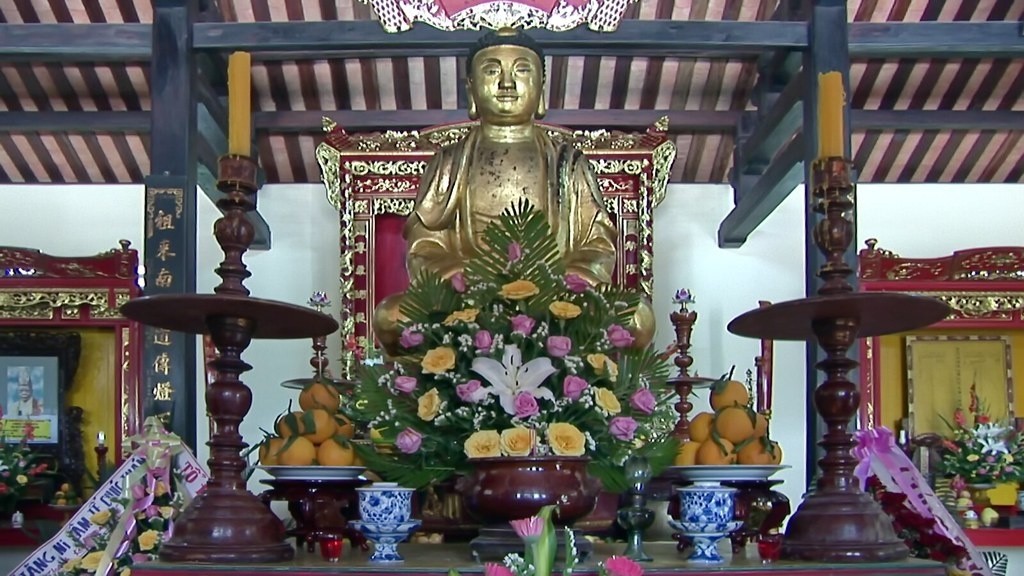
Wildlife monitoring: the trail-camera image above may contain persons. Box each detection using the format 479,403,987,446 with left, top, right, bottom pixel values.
8,372,44,416
372,27,655,388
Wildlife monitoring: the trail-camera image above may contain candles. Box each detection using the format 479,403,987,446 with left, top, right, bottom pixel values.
316,532,340,563
228,48,250,159
757,538,781,563
817,70,843,158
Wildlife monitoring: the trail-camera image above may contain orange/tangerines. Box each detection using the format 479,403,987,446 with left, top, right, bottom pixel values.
258,383,366,466
674,379,782,464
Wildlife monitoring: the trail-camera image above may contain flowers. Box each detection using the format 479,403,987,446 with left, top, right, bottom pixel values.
234,193,742,489
1,420,59,492
933,380,1024,493
469,507,646,576
62,480,183,576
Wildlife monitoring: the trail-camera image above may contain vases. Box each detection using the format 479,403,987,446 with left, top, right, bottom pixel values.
449,455,608,525
0,490,18,519
968,482,997,510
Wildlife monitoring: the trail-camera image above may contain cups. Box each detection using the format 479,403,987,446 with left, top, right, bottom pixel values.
318,535,342,563
12,511,24,528
676,481,736,522
757,537,781,563
355,483,417,523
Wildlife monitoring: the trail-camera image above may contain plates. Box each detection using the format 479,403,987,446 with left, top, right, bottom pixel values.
669,466,793,480
48,503,78,511
256,465,367,479
